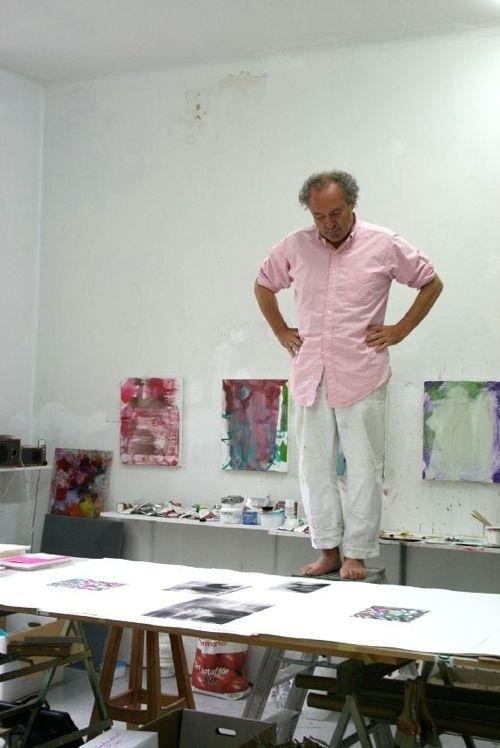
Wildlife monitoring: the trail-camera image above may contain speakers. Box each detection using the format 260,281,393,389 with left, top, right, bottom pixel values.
22,446,43,466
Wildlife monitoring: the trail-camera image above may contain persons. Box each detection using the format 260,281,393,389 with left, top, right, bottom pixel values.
258,175,443,580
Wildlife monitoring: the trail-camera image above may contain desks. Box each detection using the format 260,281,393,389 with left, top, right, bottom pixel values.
0,551,500,748
100,508,281,531
267,527,500,558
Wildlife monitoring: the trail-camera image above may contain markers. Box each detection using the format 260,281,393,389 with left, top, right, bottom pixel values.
199,518,220,522
